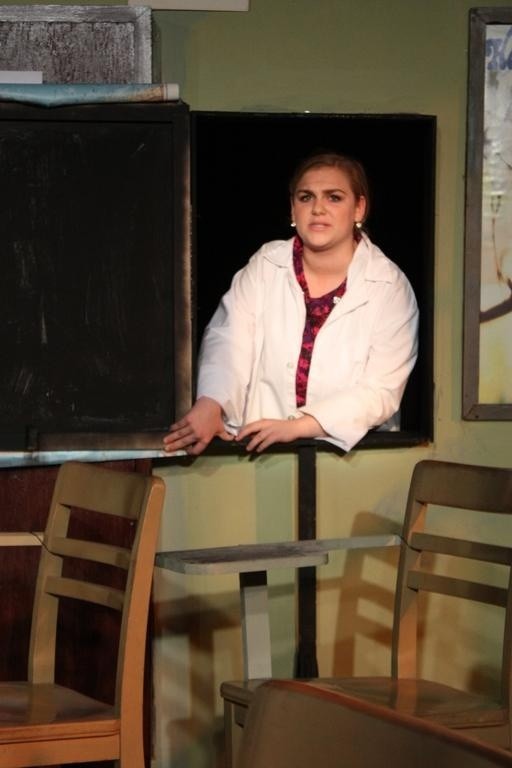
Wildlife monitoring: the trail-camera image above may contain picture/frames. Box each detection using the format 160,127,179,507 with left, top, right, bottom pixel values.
460,3,511,421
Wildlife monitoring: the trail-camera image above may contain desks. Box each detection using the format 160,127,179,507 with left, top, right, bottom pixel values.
155,529,400,679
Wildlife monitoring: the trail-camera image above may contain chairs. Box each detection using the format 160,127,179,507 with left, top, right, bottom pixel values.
219,457,512,748
232,677,512,766
1,463,166,768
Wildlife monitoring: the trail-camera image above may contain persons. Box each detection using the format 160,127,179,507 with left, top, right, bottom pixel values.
164,155,420,457
